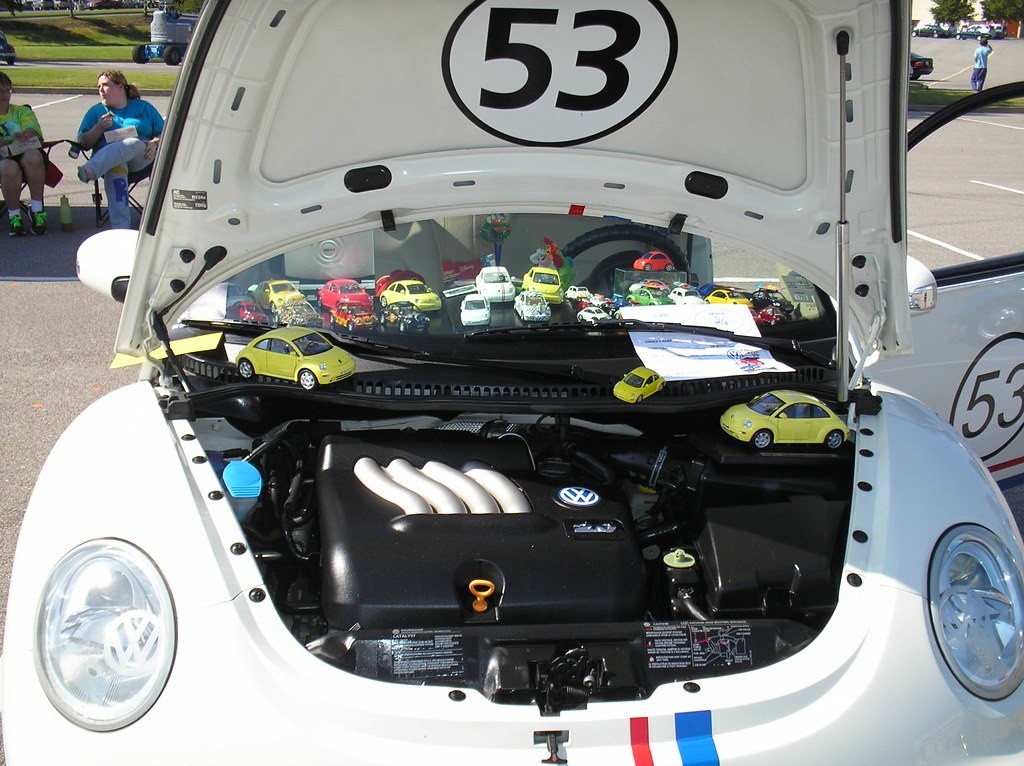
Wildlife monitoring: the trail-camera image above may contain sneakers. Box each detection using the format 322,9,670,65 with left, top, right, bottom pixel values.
27,206,48,235
8,212,27,236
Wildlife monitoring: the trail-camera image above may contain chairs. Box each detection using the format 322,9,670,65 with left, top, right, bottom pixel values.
0,104,65,216
66,139,154,229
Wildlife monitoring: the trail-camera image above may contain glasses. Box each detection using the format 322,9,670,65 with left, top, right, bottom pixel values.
0,89,13,94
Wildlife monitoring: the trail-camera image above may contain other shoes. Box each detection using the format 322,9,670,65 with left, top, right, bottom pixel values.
77,165,90,184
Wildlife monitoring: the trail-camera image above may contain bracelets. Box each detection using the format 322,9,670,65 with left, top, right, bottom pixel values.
153,141,158,150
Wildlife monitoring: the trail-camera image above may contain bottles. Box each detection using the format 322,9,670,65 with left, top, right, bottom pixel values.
59,195,72,232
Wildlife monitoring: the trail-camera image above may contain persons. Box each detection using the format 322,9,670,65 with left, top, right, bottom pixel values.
77,70,164,229
0,72,47,235
971,38,992,91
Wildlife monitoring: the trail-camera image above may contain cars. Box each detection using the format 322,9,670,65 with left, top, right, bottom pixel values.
222,250,794,336
954,24,997,42
235,326,357,394
908,52,933,81
991,22,1008,40
612,366,665,404
911,25,950,38
0,0,1023,765
719,390,850,452
